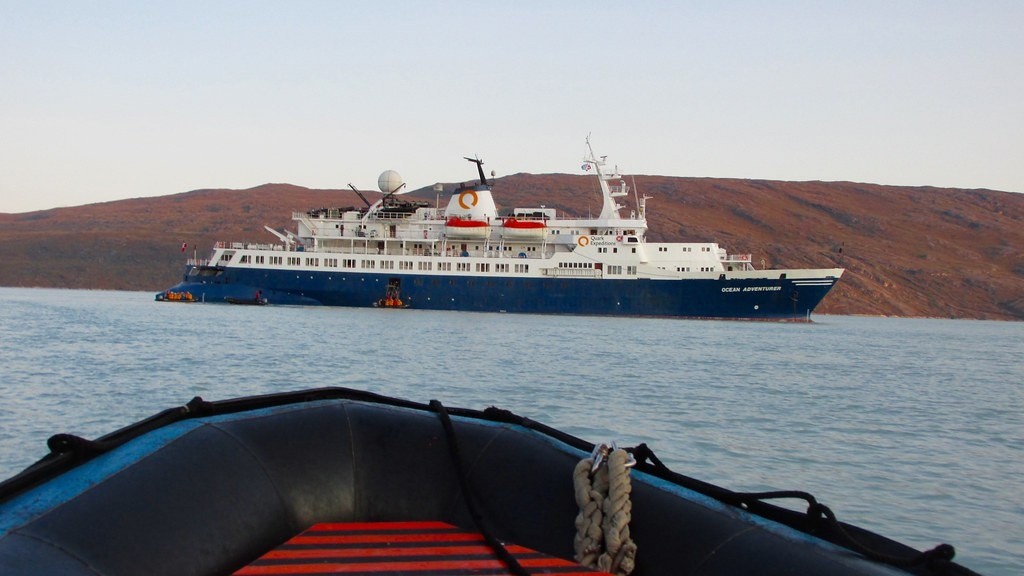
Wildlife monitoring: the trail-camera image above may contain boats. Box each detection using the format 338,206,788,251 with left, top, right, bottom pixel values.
158,297,200,303
224,295,270,305
373,301,411,309
154,136,844,324
